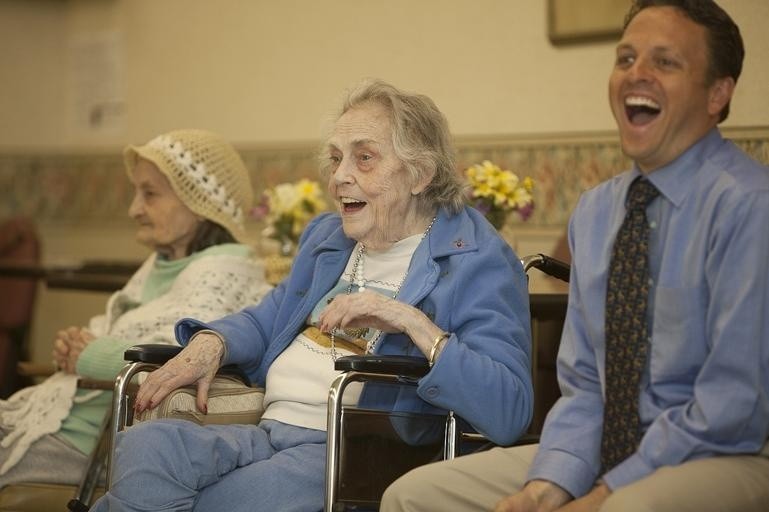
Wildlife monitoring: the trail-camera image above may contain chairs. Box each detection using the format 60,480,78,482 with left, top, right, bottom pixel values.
0,361,142,512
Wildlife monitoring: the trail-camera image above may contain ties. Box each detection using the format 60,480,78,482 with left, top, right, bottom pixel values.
594,178,658,470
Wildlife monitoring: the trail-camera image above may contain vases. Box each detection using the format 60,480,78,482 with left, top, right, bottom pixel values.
263,251,295,284
485,213,518,253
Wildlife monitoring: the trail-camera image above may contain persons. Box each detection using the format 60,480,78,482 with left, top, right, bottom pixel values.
87,79,534,512
0,130,277,496
376,1,769,512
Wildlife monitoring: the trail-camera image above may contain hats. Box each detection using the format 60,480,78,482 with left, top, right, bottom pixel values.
118,126,258,245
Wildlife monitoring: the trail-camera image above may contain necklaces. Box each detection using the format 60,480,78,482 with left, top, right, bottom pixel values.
329,228,435,365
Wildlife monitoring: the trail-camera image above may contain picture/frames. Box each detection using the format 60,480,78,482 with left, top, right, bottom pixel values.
547,0,634,46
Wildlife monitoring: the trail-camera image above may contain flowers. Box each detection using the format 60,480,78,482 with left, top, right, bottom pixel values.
461,160,536,230
250,176,329,255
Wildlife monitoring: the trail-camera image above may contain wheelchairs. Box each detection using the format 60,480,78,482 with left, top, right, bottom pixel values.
110,254,570,512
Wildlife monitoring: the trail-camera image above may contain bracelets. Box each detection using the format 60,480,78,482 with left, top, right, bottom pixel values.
429,331,450,366
